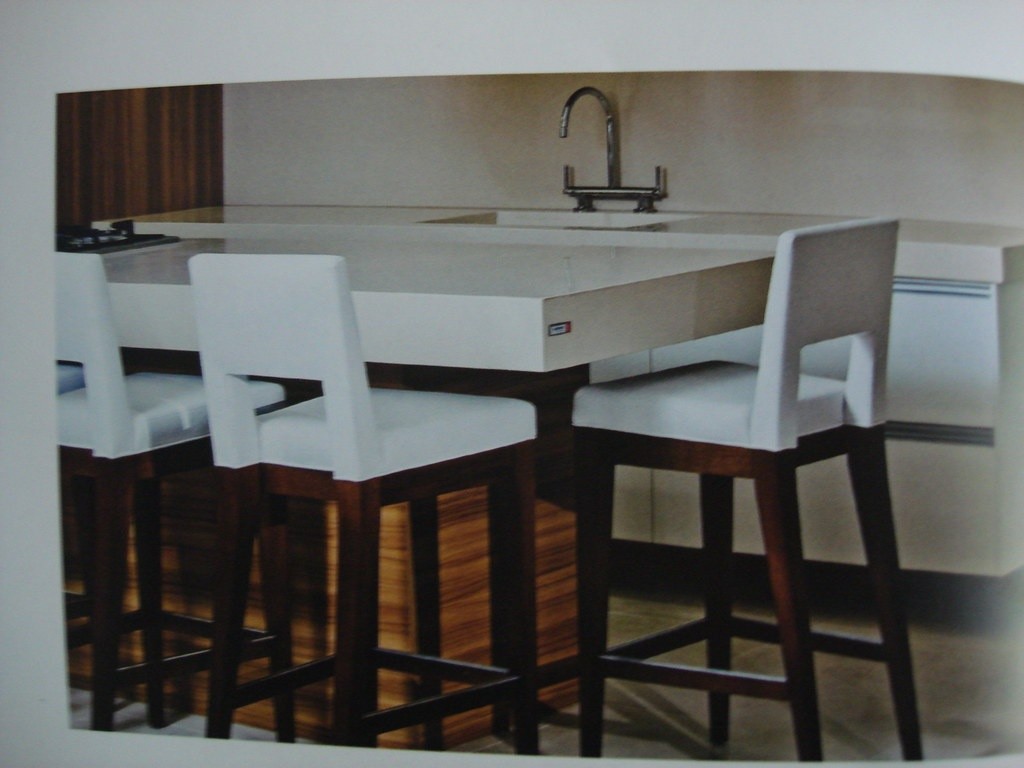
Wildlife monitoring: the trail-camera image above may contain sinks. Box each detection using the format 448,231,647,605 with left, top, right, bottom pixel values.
417,212,700,231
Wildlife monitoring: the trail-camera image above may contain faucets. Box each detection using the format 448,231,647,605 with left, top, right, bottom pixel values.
559,87,619,189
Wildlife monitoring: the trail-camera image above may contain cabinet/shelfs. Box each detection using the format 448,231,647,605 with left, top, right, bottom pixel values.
592,284,1024,640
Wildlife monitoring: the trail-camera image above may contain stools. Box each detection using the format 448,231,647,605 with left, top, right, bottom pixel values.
186,253,543,758
51,247,299,746
570,213,927,762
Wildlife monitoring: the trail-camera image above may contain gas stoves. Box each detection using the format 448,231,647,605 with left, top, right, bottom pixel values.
55,219,181,255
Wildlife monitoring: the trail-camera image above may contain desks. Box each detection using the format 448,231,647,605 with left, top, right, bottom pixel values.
98,238,777,737
